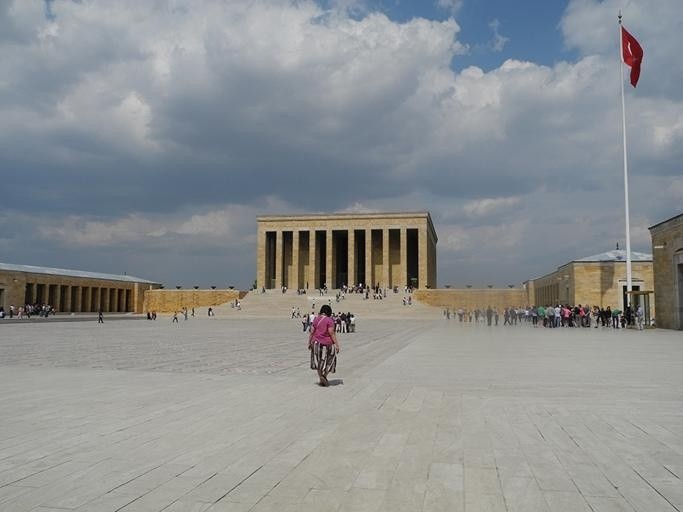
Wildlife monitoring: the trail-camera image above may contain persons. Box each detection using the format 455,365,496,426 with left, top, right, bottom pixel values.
307,305,340,384
444,302,645,330
291,306,356,334
145,297,240,324
98,311,103,323
0,298,55,320
260,280,415,307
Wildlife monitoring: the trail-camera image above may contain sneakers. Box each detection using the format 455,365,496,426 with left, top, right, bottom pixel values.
319,375,329,387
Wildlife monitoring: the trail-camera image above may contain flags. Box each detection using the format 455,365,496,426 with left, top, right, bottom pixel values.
620,24,643,87
